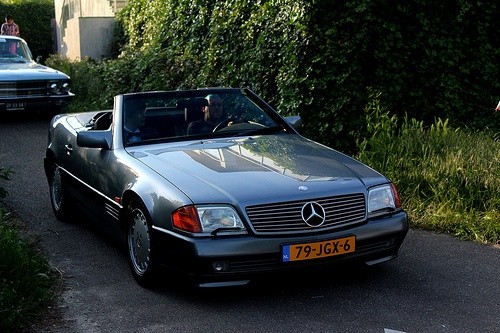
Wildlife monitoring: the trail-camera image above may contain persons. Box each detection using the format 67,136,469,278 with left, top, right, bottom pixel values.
187,95,235,135
123,101,158,145
0,14,19,55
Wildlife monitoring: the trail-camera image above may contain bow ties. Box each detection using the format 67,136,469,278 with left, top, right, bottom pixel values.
128,132,142,139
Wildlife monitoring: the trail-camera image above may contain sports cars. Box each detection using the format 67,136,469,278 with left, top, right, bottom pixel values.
42,88,413,289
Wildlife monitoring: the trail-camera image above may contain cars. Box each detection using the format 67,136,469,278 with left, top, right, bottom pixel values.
0,35,75,110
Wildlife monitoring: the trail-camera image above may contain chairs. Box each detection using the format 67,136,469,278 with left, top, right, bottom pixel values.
185,98,207,123
